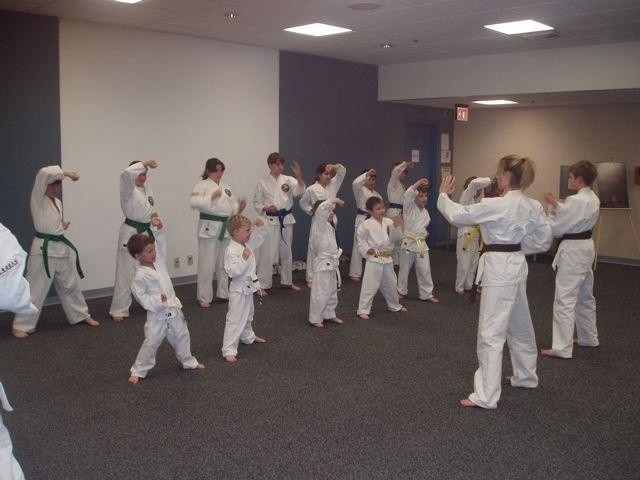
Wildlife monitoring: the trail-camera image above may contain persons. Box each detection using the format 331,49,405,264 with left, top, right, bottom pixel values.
10,165,100,340
128,216,205,385
540,161,602,360
221,216,267,362
0,222,39,480
437,153,553,411
189,157,246,309
109,160,166,323
